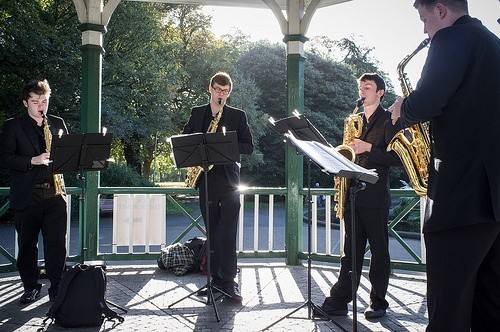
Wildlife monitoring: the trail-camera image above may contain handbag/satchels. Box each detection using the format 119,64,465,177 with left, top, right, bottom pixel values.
157,236,207,277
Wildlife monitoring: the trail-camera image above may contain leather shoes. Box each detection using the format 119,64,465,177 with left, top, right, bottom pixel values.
19,288,40,303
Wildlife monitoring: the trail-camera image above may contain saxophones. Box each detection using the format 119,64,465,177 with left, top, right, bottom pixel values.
39,110,67,195
333,95,366,220
386,37,431,197
186,97,223,190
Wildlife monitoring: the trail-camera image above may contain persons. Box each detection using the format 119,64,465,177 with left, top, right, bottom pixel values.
313,73,417,320
182,72,253,301
2,79,71,303
389,0,500,332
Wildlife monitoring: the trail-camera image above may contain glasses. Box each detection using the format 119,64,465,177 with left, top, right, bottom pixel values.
212,86,230,95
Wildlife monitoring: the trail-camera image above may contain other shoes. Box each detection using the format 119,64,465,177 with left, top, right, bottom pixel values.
198,280,223,296
313,302,348,317
364,306,387,317
221,286,243,301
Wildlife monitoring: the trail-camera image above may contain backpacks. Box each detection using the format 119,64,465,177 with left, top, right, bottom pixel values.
43,263,124,328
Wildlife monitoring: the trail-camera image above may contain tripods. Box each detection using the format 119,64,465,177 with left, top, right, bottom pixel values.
168,130,239,322
262,114,348,332
